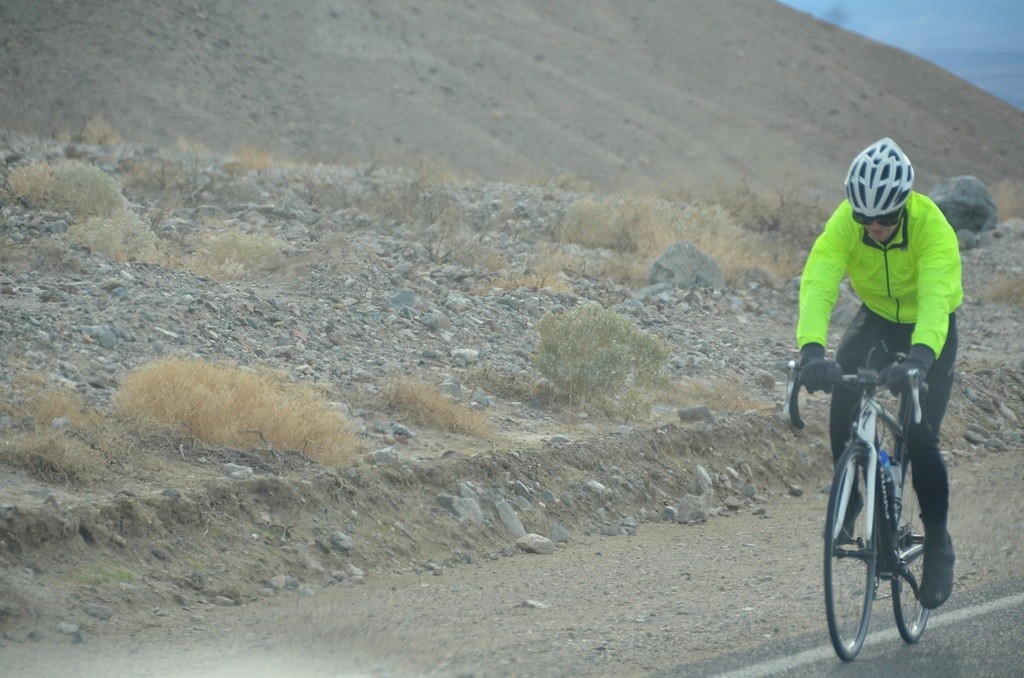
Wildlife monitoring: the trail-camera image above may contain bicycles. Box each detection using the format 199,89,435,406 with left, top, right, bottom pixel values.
783,352,931,664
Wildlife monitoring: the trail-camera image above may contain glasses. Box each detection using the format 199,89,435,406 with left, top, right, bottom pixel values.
852,208,901,226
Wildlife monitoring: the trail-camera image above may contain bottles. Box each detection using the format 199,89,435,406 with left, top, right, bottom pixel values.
880,450,892,481
888,456,902,522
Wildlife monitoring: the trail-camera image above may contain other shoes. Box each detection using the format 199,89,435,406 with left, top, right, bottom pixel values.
823,488,864,546
920,533,953,613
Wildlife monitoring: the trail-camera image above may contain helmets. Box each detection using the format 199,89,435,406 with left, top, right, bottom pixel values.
844,138,914,217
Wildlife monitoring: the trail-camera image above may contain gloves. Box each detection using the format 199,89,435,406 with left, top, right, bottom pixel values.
800,356,842,394
879,358,926,399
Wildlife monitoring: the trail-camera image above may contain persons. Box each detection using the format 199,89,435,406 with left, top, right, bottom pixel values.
796,138,964,608
